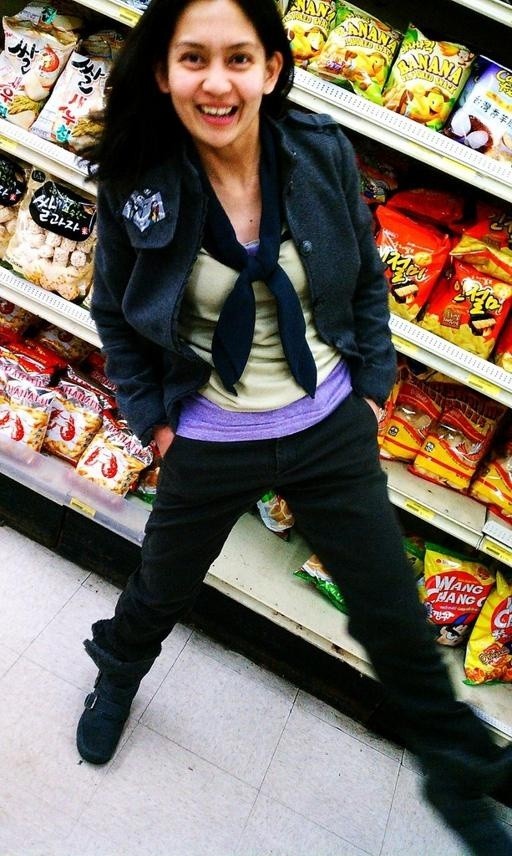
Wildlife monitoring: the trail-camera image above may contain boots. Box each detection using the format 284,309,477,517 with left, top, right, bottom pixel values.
76,620,162,765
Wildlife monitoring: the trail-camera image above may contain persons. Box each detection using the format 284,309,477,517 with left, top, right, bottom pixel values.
68,1,512,856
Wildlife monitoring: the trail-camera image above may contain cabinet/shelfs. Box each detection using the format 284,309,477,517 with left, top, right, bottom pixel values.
1,1,512,802
